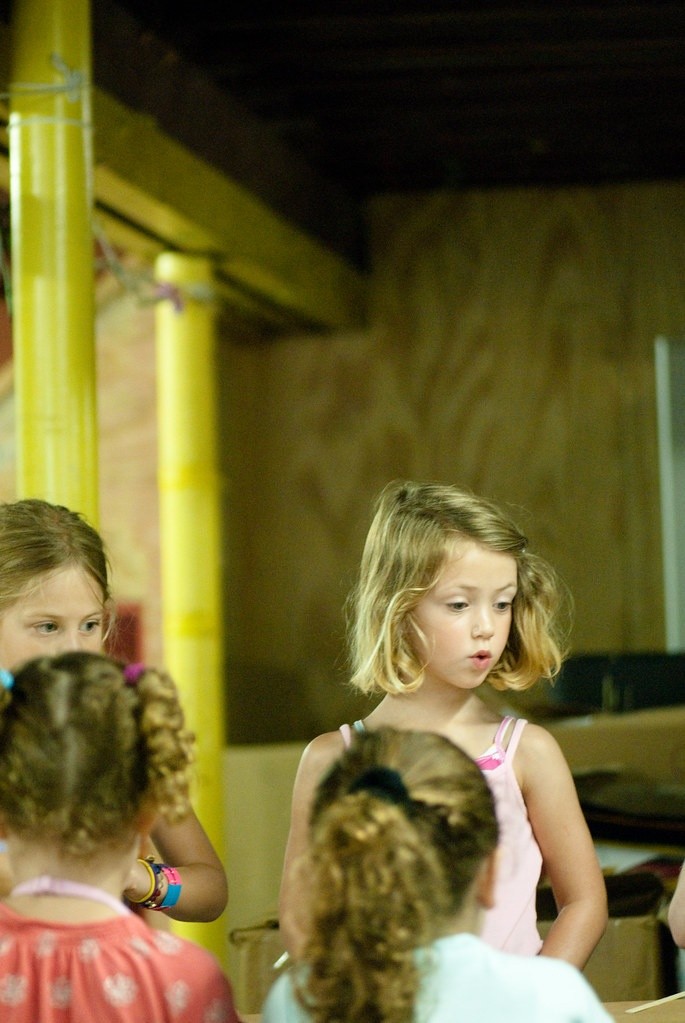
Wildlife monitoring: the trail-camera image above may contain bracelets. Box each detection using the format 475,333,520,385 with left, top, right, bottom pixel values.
129,854,184,912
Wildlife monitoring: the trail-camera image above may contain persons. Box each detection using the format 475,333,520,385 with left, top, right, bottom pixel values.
0,649,243,1023
281,479,609,972
0,497,229,923
261,725,616,1023
